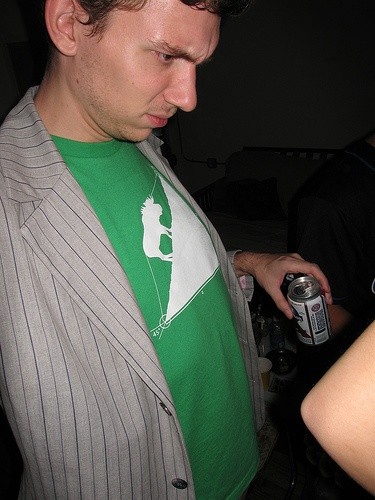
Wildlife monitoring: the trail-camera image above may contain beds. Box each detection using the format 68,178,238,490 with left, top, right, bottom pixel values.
209,146,341,252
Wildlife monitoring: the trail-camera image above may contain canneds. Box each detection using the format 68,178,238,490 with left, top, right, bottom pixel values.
287,276,332,346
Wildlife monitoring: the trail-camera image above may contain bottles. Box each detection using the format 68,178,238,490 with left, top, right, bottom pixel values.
262,329,300,392
271,311,280,329
255,304,262,319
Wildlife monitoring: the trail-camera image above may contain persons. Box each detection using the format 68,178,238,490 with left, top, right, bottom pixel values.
300,312,374,496
1,0,333,500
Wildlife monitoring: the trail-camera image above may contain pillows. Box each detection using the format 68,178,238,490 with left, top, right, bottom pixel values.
224,150,291,181
276,159,331,221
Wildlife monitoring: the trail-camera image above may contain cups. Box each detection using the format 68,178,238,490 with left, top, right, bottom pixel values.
258,357,272,402
